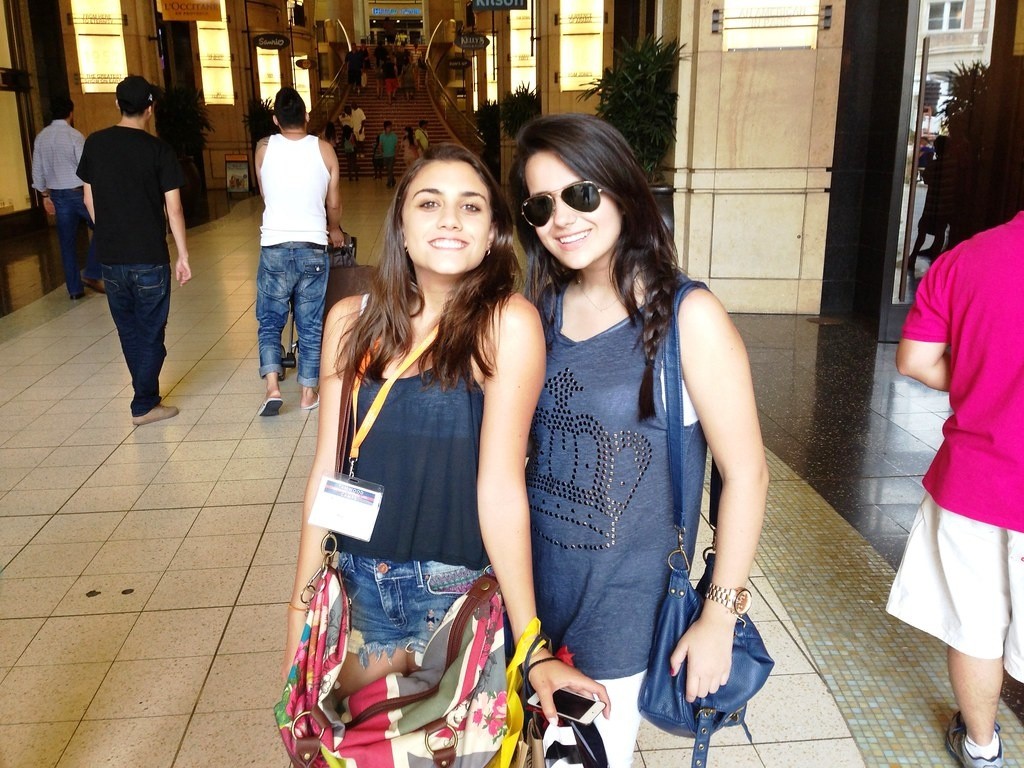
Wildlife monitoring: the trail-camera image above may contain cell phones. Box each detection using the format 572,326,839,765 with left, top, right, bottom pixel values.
527,688,607,726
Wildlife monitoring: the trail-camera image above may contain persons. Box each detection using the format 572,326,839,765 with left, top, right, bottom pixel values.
270,142,612,768
31,96,106,301
907,135,960,272
506,112,775,768
74,74,193,425
254,86,346,417
322,101,431,189
344,17,423,106
885,208,1024,768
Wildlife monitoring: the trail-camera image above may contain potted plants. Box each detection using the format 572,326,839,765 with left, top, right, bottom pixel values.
474,98,500,182
577,34,688,239
150,81,216,219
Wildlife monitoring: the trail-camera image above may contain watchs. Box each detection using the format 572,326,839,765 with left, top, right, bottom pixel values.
705,582,753,615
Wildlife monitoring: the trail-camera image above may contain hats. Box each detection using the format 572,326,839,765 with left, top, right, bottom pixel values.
116,75,162,112
419,120,428,128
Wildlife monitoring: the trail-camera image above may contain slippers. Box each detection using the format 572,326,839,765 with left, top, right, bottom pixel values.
300,390,319,410
258,397,283,416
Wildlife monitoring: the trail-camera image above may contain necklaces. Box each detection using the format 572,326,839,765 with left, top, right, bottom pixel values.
574,270,643,313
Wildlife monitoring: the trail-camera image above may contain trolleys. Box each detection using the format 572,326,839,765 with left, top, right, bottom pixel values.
278,230,357,383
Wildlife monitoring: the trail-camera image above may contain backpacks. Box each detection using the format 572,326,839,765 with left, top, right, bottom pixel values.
345,137,354,153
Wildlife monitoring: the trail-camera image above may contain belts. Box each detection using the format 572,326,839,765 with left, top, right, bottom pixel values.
262,242,333,252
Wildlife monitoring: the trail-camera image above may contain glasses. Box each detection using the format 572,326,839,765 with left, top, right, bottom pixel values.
521,180,630,227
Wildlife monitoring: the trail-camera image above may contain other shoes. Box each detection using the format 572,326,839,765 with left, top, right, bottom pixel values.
70,275,106,300
357,153,365,157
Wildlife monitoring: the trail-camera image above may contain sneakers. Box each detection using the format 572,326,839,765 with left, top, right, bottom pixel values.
133,403,178,425
944,710,1003,768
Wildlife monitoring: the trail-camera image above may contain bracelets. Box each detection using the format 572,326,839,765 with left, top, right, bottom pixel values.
526,645,575,679
288,603,306,612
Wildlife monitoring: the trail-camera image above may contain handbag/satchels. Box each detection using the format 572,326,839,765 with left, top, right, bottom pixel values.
361,72,367,87
373,158,384,169
637,550,775,739
272,530,508,767
510,631,609,768
